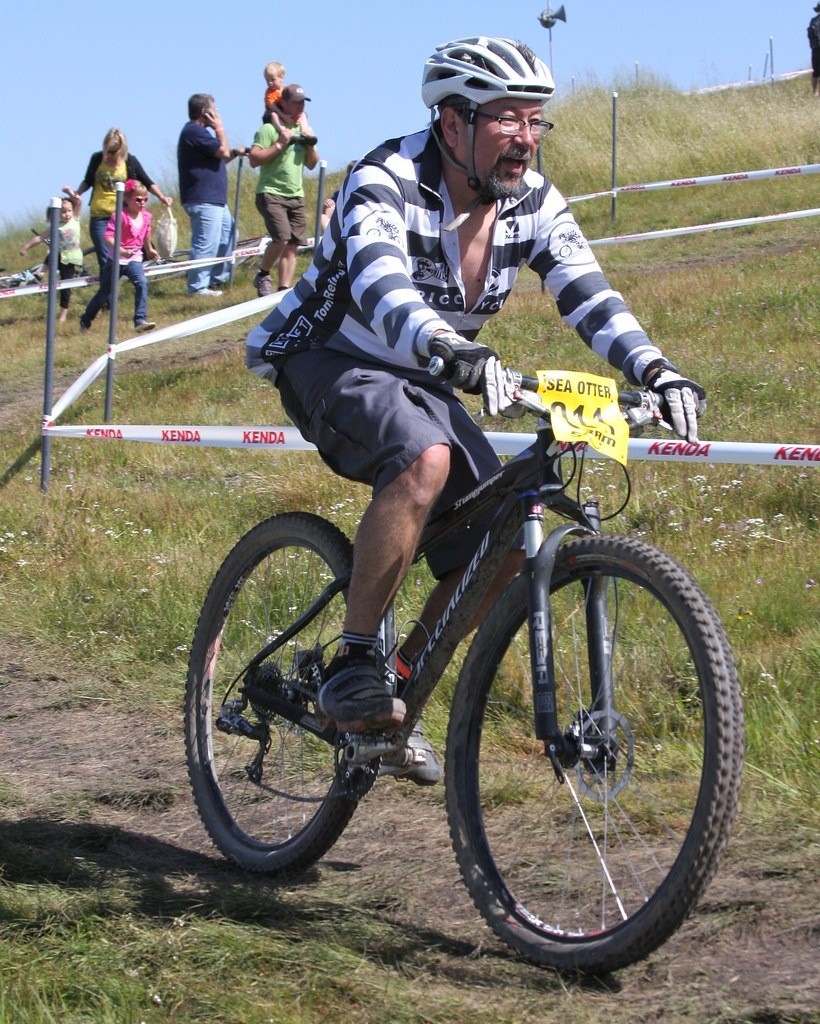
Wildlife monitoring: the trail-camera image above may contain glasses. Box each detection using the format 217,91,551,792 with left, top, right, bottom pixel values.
454,108,554,141
126,196,148,203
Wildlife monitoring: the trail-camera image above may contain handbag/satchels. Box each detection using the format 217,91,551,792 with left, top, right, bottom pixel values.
156,206,177,257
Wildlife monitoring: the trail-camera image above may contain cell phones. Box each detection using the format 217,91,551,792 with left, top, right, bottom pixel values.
201,109,212,119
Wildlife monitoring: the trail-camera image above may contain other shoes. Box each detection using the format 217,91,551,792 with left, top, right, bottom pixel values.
188,288,222,297
134,321,156,331
80,317,87,335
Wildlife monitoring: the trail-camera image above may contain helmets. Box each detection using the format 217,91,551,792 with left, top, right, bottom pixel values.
421,37,556,108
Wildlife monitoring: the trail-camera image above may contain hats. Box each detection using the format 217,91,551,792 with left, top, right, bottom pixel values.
282,84,311,102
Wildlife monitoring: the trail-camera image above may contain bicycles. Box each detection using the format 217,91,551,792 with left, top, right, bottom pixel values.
182,357,748,976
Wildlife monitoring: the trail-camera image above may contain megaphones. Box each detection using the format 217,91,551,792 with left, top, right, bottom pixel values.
545,5,566,22
540,9,556,28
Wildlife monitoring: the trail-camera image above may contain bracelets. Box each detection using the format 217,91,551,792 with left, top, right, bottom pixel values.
214,126,224,132
232,148,238,157
275,141,283,150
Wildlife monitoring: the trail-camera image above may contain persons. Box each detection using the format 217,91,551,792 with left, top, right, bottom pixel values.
79,180,155,335
249,84,319,296
19,186,83,324
247,38,706,782
73,127,173,308
807,3,820,97
262,62,318,147
321,160,358,233
177,94,250,297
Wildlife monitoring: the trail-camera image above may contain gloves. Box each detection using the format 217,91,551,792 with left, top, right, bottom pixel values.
429,330,506,416
643,365,707,444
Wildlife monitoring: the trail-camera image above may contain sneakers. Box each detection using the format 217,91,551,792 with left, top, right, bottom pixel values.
253,273,272,297
314,663,405,732
380,717,439,786
